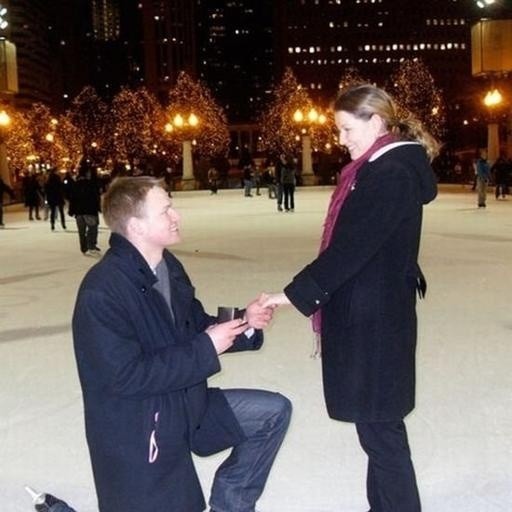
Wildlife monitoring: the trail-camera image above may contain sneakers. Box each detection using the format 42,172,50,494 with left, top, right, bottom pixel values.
87,244,100,251
35,494,75,511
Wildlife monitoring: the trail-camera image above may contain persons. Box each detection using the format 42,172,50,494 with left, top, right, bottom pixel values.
208,164,221,194
1,157,101,256
161,166,173,198
453,152,512,207
241,154,297,212
34,173,293,512
258,82,438,512
